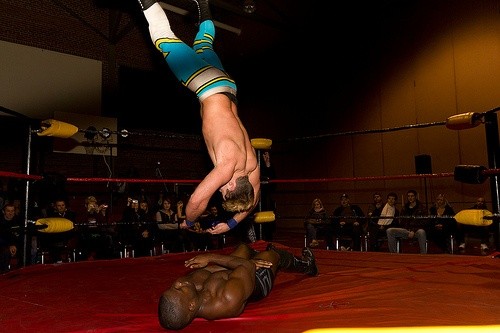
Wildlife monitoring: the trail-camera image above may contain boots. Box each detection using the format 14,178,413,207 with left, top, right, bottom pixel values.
293,246,319,276
266,242,274,251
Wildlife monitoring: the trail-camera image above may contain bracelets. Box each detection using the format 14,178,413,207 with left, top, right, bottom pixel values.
227,217,238,229
185,220,193,226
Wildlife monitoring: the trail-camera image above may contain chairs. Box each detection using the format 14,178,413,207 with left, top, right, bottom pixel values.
303,230,459,256
7,234,225,270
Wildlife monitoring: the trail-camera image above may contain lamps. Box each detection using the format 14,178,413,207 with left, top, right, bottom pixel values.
99,126,111,141
83,125,97,141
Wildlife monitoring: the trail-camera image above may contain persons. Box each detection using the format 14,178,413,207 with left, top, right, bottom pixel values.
0,183,121,274
303,198,330,249
158,243,318,330
326,193,367,251
139,1,262,234
427,193,466,252
114,189,227,257
386,191,428,254
365,192,399,252
455,198,491,251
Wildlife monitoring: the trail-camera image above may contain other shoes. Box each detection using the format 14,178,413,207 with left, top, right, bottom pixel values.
312,240,318,246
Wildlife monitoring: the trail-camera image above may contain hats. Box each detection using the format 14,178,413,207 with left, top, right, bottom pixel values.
340,193,350,199
